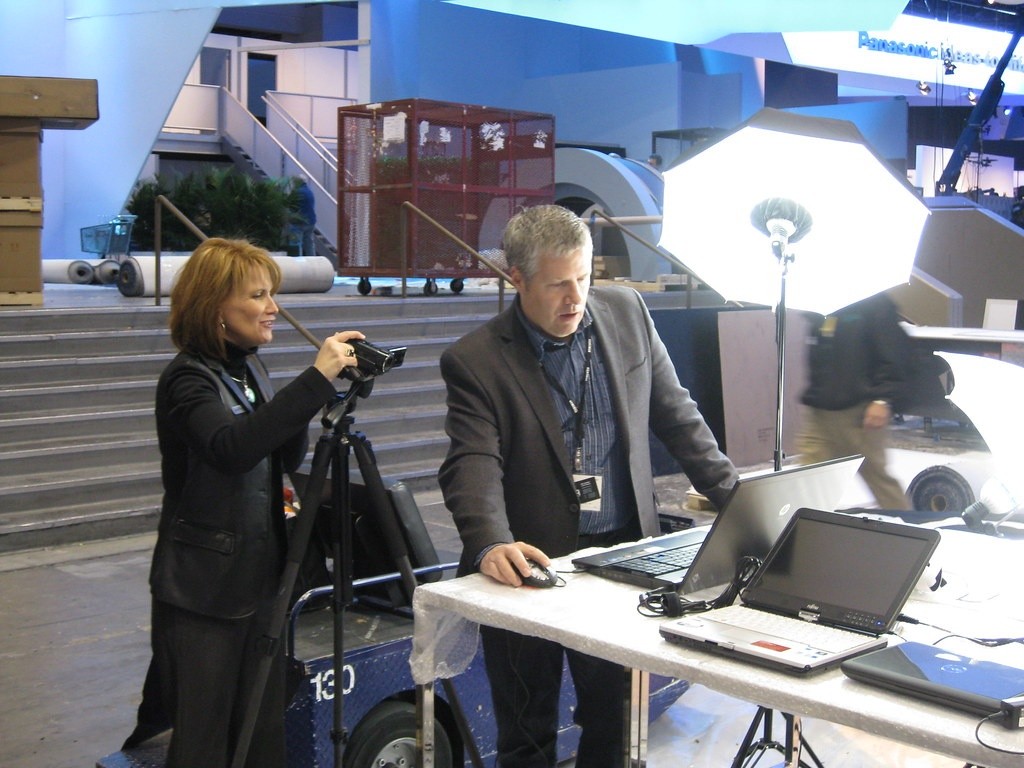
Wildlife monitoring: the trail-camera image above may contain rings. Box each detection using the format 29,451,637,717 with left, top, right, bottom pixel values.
349,350,354,357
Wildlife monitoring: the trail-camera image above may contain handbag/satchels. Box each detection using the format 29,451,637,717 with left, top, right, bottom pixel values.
282,489,333,614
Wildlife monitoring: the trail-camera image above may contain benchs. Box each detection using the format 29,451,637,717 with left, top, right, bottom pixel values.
298,466,464,605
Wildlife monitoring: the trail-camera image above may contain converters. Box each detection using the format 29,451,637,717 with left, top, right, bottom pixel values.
1002,696,1024,730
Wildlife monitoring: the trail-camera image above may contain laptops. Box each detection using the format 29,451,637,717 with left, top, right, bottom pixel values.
658,509,940,678
840,642,1024,719
571,453,864,593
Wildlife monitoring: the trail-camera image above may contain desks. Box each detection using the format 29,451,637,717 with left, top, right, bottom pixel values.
411,525,1024,768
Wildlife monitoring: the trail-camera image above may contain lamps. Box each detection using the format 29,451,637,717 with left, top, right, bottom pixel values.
917,81,931,96
967,87,978,105
945,60,957,74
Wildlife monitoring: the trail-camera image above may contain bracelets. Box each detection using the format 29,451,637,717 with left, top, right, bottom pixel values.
873,400,887,405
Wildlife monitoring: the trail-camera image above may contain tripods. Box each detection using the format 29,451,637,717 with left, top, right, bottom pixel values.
231,366,487,768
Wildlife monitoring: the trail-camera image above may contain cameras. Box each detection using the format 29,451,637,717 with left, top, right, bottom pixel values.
342,338,407,375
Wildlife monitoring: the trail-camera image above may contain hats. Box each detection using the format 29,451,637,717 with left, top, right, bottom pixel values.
291,173,308,184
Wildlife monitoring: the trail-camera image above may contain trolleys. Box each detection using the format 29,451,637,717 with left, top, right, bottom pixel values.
80,213,137,260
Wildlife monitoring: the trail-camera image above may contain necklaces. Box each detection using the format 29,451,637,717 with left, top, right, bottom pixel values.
230,367,255,403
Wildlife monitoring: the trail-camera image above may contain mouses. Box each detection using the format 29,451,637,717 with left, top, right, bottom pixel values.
509,560,558,588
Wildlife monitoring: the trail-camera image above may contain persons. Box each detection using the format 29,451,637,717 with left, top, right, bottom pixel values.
442,205,739,768
122,237,365,767
799,292,912,510
284,173,316,256
